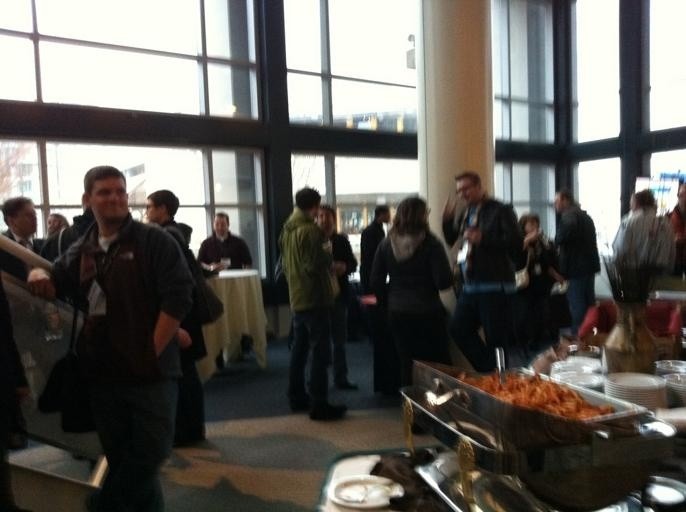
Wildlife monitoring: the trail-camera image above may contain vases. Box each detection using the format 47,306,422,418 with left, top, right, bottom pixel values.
602,301,659,374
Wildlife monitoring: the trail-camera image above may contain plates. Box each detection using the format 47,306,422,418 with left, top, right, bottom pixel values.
637,474,686,507
323,474,406,509
546,350,686,430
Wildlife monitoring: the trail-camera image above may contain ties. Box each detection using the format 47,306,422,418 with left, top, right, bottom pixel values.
20,240,30,272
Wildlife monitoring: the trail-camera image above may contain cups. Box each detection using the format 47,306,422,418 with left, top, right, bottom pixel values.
220,257,231,270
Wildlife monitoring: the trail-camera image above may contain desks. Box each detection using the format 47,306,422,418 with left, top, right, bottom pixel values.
196,269,269,386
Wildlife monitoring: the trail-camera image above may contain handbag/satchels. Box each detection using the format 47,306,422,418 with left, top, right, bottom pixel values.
39,354,121,424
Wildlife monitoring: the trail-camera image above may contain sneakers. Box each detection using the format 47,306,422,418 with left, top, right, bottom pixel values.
310,402,346,420
289,394,310,410
